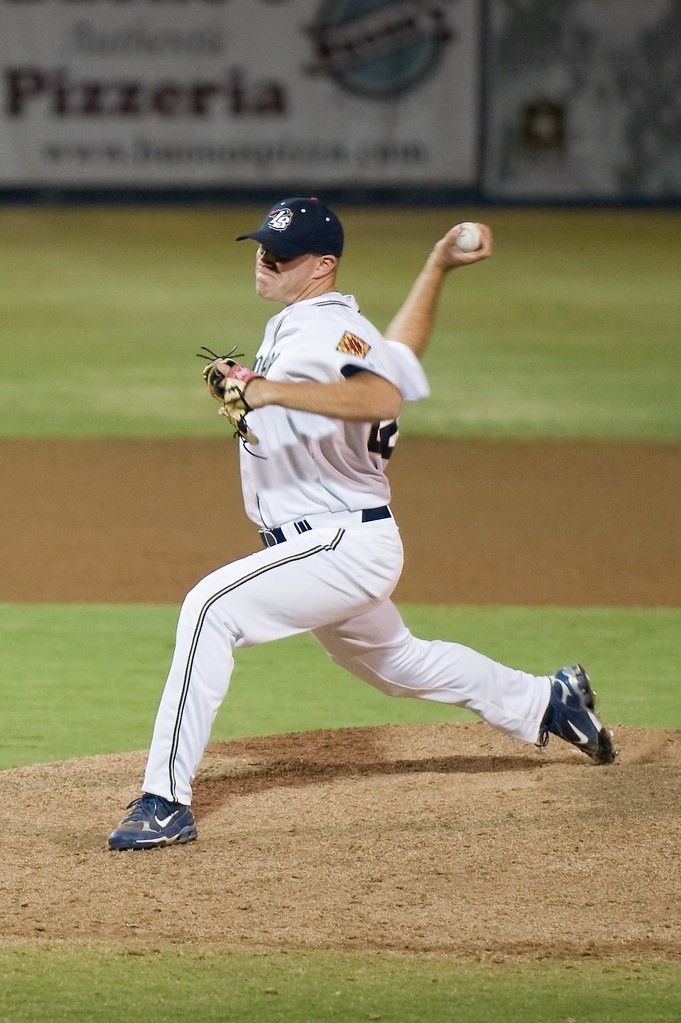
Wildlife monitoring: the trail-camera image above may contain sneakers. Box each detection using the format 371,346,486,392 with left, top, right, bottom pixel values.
109,793,198,852
535,664,615,763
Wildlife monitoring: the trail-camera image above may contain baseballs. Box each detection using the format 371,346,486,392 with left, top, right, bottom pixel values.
455,222,482,252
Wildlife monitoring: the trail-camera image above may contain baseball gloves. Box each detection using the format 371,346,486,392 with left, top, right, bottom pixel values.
201,356,263,448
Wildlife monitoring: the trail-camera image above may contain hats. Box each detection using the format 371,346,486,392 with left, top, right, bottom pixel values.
236,197,344,259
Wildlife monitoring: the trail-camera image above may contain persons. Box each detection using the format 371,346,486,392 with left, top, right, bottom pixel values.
108,196,617,849
